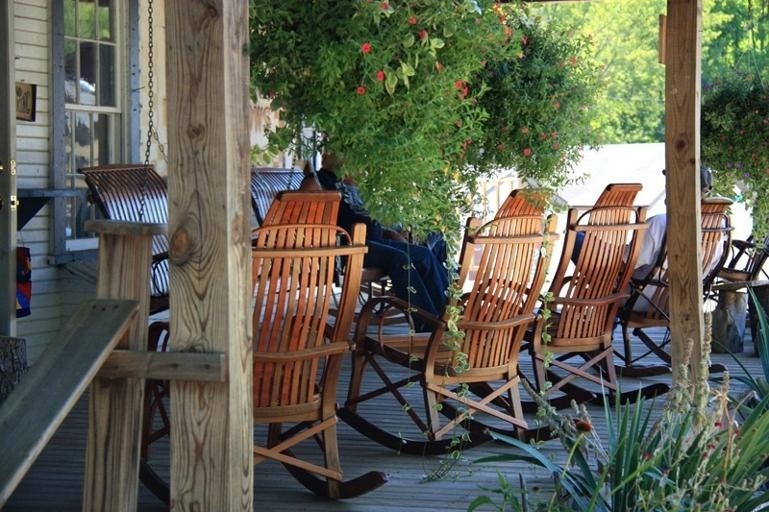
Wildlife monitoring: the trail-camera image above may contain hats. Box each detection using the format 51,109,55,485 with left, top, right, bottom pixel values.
662,167,712,187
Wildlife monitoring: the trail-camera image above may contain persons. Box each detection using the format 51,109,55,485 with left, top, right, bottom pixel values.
296,138,451,332
568,164,723,317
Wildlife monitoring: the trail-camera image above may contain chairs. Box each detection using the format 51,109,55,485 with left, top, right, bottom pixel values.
273,187,591,455
456,183,669,413
705,218,769,305
139,192,389,512
617,197,733,377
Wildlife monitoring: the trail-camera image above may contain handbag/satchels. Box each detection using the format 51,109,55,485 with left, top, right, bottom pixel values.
16,246,32,318
